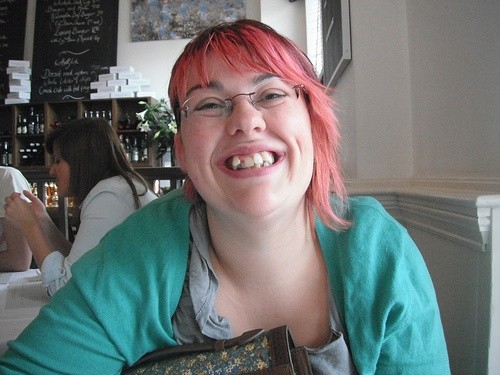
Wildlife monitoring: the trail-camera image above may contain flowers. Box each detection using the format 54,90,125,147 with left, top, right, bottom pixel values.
135,97,177,145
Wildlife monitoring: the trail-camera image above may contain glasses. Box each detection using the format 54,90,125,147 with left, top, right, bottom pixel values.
176,77,305,122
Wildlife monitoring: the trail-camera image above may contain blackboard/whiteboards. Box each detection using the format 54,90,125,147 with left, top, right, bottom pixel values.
31,0,118,99
0,0,27,104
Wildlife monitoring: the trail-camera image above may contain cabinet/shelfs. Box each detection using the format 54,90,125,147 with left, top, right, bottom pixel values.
0,96,159,244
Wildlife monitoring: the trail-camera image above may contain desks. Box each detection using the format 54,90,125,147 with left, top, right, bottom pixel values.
0,269,52,358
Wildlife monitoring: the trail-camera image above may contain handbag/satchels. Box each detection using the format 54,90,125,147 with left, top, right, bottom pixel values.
119,324,314,375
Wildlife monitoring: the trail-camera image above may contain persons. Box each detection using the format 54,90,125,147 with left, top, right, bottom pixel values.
0,19,452,375
0,118,162,301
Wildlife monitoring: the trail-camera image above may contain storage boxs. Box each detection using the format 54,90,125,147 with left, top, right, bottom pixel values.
90,66,156,98
5,61,31,104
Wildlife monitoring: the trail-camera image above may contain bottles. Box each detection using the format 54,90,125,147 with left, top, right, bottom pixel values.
29,183,37,198
140,134,148,162
41,114,45,134
84,111,88,118
102,111,106,119
118,113,137,130
131,137,139,162
19,148,31,166
22,118,27,134
120,135,131,162
3,142,12,167
35,114,41,134
95,110,100,118
17,115,21,134
30,143,45,157
27,107,35,134
107,111,112,126
45,182,58,207
89,111,94,118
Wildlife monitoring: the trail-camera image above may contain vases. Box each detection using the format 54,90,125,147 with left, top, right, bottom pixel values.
171,145,179,166
